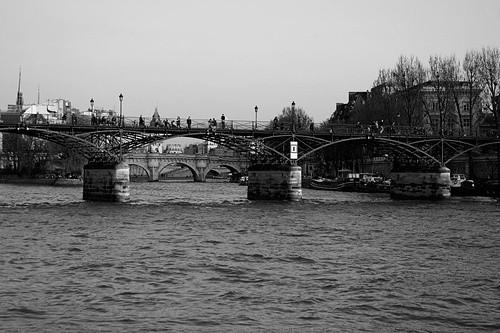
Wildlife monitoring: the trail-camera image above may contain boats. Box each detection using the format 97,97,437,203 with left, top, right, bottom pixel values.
302,163,500,202
0,173,59,186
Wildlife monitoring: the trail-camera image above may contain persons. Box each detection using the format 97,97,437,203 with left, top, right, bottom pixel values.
62,113,226,130
275,117,498,138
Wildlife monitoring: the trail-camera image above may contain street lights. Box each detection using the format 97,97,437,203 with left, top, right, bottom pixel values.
90,98,95,126
254,105,258,130
440,109,444,136
291,101,296,132
396,112,401,135
119,93,124,127
